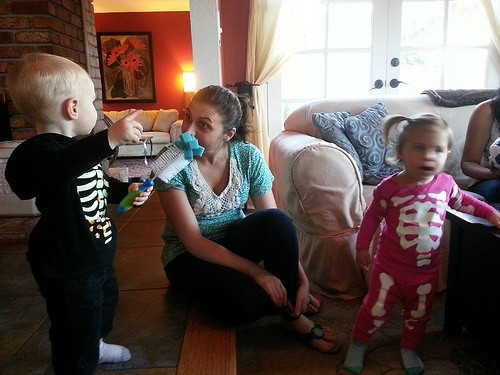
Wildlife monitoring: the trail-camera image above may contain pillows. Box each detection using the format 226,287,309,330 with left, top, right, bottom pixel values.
152,109,177,132
348,103,405,186
311,111,364,182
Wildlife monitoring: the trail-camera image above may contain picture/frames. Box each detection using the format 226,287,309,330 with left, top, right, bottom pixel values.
97,32,156,104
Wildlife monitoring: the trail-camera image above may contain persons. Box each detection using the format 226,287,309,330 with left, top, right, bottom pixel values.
459,86,500,228
344,114,500,374
5,50,155,375
154,85,345,354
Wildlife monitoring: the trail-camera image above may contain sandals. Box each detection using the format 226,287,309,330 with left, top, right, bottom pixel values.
306,294,327,315
293,314,342,352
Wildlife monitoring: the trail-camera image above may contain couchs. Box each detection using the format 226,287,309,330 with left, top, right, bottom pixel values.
268,88,500,344
103,109,183,156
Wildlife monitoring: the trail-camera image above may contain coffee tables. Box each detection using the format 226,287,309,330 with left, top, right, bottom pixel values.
140,137,153,165
442,201,500,375
